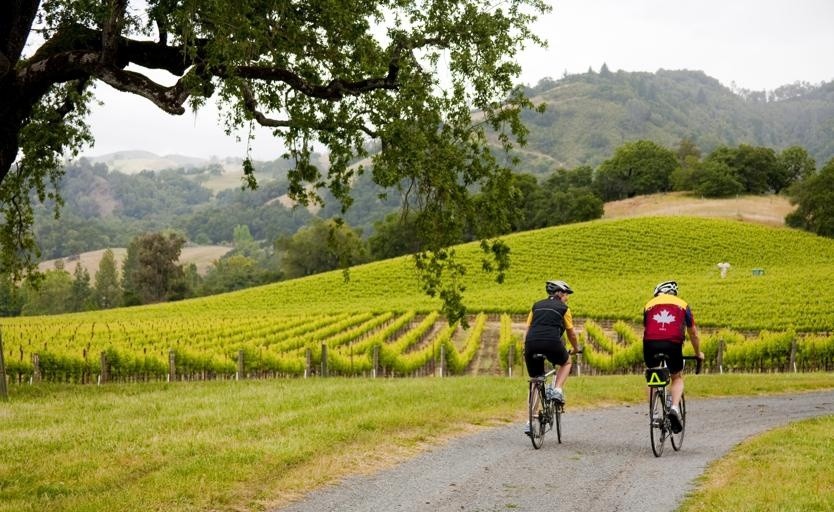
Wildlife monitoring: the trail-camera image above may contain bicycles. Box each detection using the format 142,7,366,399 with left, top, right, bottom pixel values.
526,347,582,449
646,351,702,456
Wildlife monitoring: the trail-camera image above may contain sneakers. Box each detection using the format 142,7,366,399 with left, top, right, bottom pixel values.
549,389,564,404
524,423,539,438
669,405,683,434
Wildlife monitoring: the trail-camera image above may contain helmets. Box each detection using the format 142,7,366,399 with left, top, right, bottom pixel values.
653,281,678,296
545,280,573,294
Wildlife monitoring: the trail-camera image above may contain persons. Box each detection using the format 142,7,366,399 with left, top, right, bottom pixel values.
639,279,707,435
716,258,730,280
520,279,580,444
